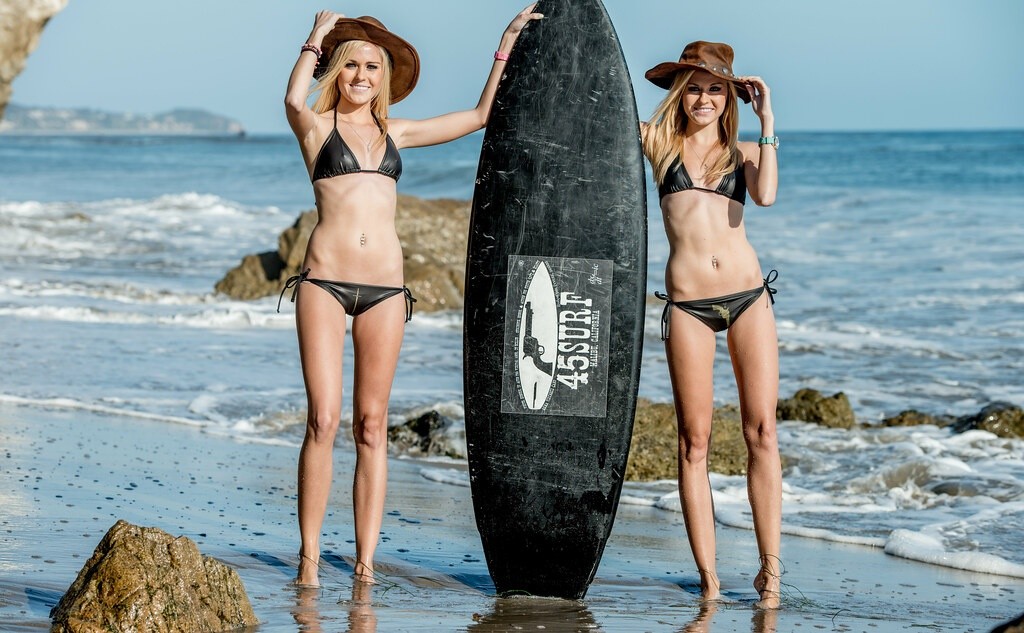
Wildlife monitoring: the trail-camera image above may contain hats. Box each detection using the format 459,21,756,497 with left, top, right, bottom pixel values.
309,15,420,105
645,41,755,103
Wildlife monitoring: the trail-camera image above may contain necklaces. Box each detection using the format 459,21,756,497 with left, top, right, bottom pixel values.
687,139,712,169
337,112,376,153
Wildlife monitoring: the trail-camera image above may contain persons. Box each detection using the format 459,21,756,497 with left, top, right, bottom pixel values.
284,3,544,588
637,41,783,615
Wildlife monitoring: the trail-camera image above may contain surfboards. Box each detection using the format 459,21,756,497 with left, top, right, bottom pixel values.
462,1,647,602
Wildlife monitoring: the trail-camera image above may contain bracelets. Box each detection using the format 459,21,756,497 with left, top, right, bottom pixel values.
300,42,323,65
493,51,510,62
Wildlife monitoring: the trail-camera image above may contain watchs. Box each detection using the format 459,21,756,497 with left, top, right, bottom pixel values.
758,136,779,150
